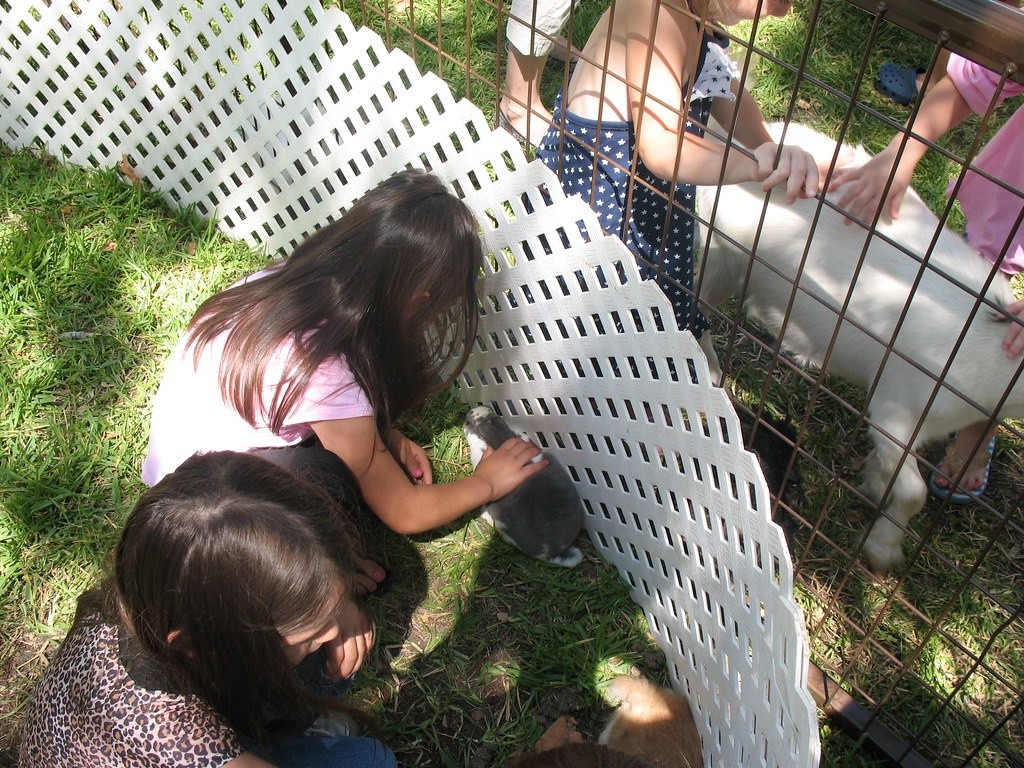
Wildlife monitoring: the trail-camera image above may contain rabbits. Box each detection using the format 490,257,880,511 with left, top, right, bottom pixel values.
461,404,586,567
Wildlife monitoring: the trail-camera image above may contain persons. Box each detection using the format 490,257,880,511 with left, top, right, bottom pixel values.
139,169,548,599
17,450,397,768
502,0,1024,505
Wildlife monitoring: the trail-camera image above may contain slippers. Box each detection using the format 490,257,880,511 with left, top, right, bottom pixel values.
929,431,996,504
352,553,392,597
875,64,927,105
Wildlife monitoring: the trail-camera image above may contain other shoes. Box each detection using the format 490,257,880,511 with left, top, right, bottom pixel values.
300,708,358,738
499,97,547,152
504,39,581,73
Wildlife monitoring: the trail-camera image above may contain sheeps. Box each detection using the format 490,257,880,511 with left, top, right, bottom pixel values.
691,43,1024,574
